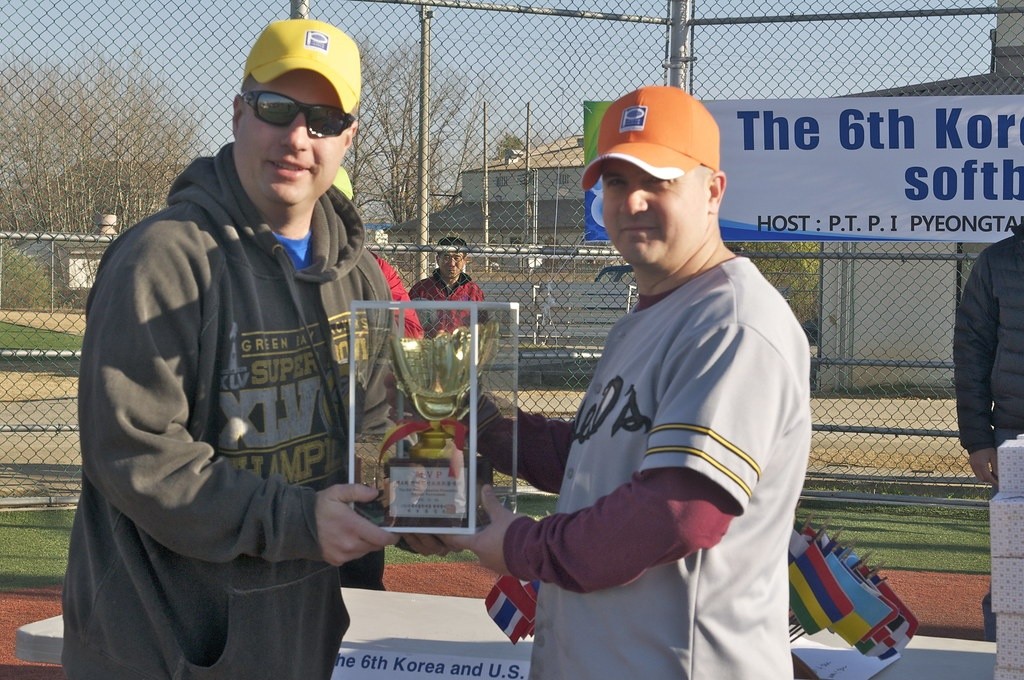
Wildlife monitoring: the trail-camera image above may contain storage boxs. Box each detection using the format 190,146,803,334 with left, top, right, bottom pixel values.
988,433,1024,680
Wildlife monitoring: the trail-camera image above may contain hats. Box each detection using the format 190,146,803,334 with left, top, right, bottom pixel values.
580,86,720,192
240,20,361,113
333,165,354,201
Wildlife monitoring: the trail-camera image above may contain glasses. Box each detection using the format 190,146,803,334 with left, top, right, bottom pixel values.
240,91,357,137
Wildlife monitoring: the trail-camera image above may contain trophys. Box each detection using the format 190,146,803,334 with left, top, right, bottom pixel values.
375,321,495,527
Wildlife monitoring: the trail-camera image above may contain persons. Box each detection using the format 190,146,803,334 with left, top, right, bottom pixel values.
384,84,812,680
62,19,446,680
954,227,1024,640
331,166,486,341
